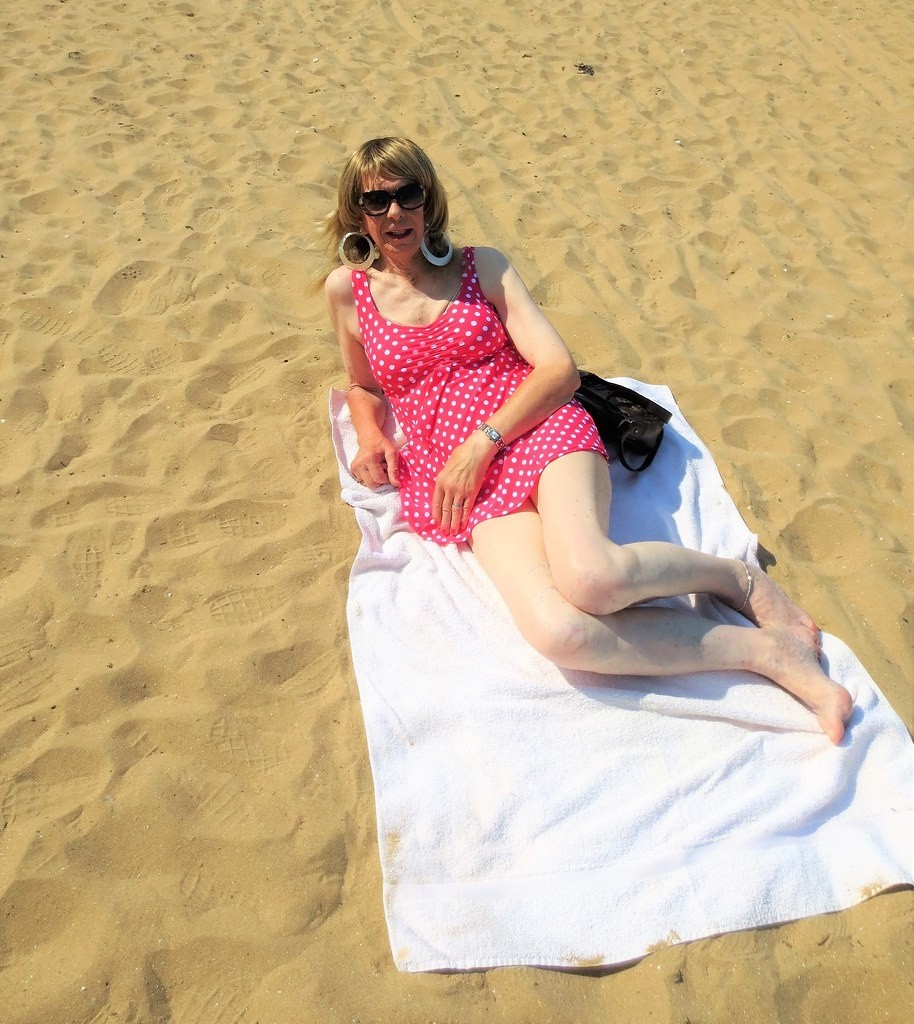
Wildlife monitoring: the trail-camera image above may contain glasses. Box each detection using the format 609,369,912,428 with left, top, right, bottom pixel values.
355,183,428,216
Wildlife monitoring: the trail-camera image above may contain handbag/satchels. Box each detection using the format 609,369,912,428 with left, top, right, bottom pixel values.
574,369,673,472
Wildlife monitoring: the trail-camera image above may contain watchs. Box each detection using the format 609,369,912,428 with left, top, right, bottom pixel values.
478,422,506,452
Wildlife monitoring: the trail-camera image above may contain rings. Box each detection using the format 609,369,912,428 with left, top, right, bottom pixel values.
453,503,463,508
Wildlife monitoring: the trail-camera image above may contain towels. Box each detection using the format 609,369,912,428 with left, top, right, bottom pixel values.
327,377,914,975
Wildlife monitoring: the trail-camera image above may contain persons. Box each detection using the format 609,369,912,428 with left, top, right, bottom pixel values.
315,138,852,747
